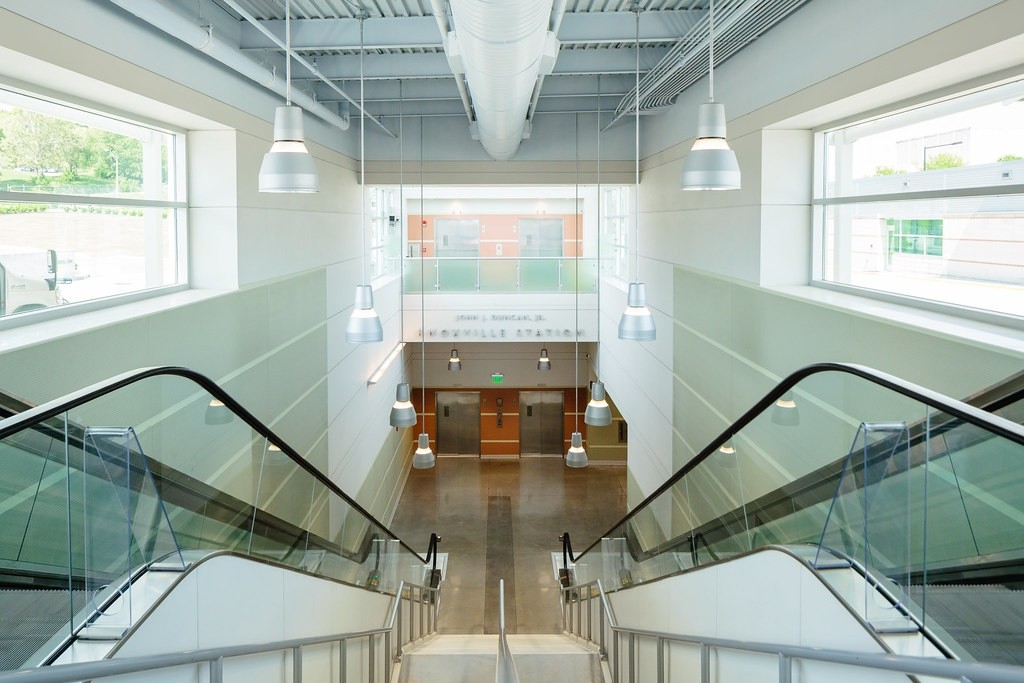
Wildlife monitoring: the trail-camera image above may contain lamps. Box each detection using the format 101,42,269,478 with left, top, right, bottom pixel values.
538,342,550,371
447,343,461,371
256,0,315,195
681,0,742,192
412,116,436,469
567,113,589,468
583,71,612,427
368,342,407,386
618,3,657,342
389,76,417,428
345,17,384,346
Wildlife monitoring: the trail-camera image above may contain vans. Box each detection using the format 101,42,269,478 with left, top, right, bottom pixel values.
0,250,73,318
